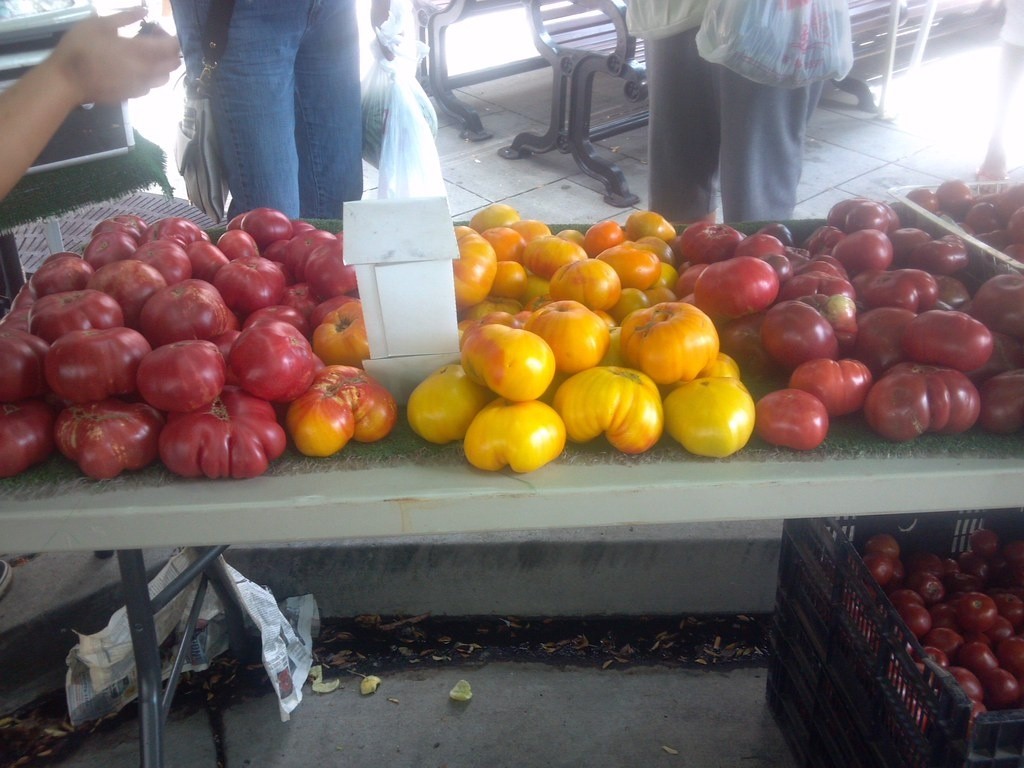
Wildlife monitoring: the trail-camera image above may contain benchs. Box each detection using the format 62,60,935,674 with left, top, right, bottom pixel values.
498,1,1007,210
407,0,527,142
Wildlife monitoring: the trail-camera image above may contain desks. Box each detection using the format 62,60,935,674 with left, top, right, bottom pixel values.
0,219,1024,768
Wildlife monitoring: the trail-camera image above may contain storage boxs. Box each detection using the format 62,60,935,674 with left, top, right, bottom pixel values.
765,507,1024,768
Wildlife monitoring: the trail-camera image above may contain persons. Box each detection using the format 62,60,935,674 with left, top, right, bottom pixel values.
0,5,183,205
170,1,395,222
643,0,827,227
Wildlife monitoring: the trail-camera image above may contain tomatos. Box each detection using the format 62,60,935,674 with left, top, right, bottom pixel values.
815,527,1024,762
0,206,399,480
671,174,1024,454
284,202,756,471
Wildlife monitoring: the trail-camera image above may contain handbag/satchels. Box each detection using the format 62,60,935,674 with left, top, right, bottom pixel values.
360,12,440,169
624,0,857,88
174,76,230,223
